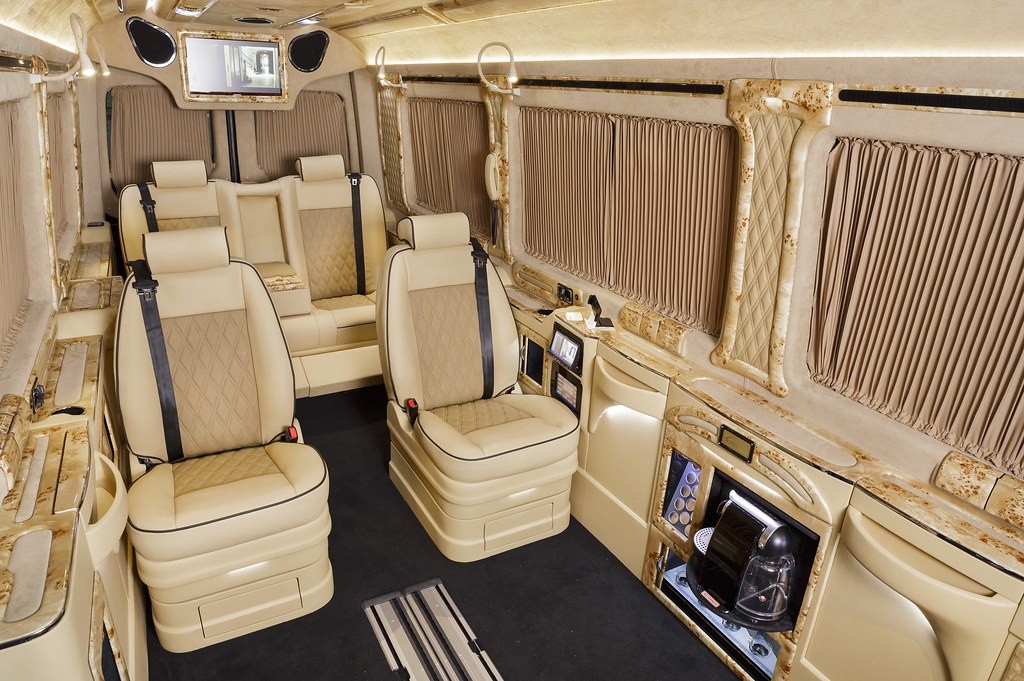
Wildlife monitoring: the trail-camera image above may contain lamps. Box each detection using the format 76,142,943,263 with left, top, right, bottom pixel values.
375,46,406,92
477,42,519,97
64,36,109,81
29,13,94,85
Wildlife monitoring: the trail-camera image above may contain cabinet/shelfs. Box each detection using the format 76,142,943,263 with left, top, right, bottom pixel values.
644,380,1024,681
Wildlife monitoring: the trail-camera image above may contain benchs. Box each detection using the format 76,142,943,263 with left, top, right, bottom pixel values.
117,155,387,399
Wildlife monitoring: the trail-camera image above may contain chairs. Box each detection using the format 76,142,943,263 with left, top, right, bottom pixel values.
112,226,334,652
375,212,580,564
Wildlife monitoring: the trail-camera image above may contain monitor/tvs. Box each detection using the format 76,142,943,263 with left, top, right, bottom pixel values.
177,29,290,104
